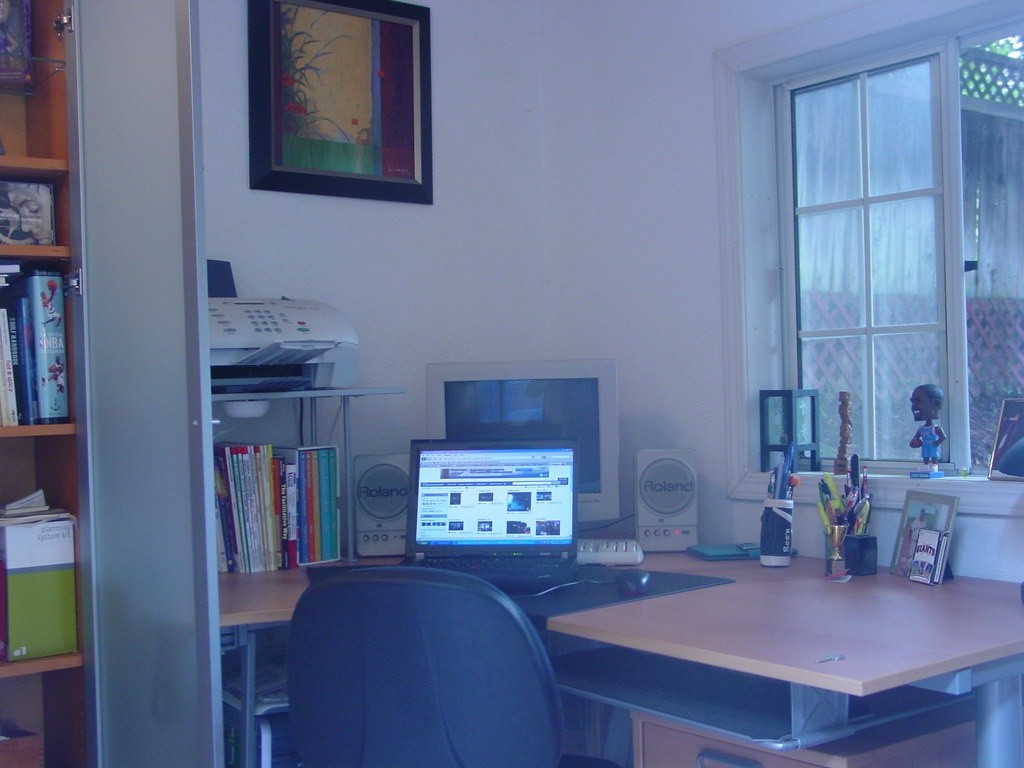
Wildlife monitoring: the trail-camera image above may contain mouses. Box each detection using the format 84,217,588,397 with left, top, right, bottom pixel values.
614,566,653,598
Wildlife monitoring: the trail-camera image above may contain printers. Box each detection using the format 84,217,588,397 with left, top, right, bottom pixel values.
206,256,360,392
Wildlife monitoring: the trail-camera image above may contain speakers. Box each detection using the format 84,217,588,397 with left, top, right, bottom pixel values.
353,451,408,556
634,446,698,551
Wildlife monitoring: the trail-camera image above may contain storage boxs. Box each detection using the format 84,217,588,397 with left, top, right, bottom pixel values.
0,520,78,663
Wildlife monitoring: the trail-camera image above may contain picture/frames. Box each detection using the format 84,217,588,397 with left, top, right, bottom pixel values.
888,489,960,582
247,0,433,205
988,397,1024,482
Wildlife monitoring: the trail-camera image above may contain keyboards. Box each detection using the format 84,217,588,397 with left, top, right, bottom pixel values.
397,555,577,591
578,536,642,567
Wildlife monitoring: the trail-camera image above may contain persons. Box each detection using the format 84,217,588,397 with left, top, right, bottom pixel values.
8,183,52,245
42,356,65,394
908,508,927,557
910,384,946,472
40,288,62,327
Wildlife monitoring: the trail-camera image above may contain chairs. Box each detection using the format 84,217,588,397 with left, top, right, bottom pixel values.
285,564,619,768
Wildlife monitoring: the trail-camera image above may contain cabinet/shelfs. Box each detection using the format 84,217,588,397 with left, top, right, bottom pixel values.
0,0,87,768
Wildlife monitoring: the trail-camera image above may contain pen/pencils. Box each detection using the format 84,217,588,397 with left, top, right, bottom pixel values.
768,441,798,499
813,454,873,536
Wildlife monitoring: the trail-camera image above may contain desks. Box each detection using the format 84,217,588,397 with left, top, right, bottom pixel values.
218,531,1024,768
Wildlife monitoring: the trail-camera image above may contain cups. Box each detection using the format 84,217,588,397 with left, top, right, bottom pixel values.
760,499,794,566
826,524,848,561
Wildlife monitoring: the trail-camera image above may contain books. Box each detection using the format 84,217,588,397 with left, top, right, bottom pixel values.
0,269,68,427
213,440,337,574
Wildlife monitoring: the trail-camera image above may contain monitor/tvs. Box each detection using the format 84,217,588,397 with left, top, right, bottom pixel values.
402,439,577,559
423,358,619,525
